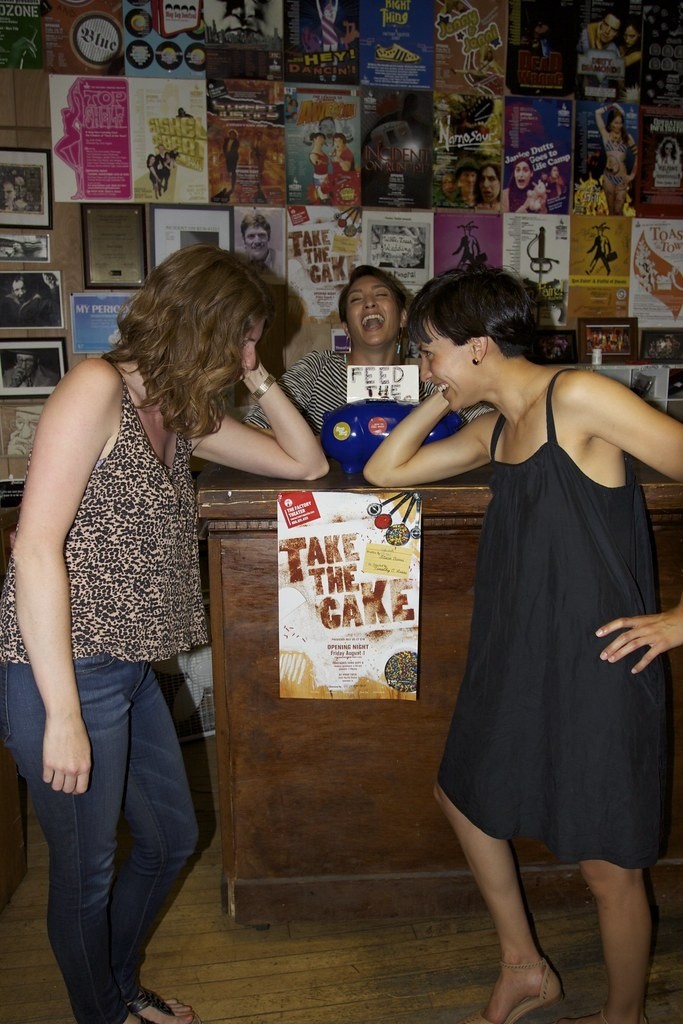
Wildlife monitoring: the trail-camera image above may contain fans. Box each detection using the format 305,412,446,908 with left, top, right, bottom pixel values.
151,589,216,742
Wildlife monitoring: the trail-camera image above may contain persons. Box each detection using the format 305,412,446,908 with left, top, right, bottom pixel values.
306,117,355,205
222,129,240,193
364,265,683,1024
648,335,680,359
0,240,43,260
0,273,61,327
521,11,642,102
4,38,37,68
316,0,338,83
0,165,42,213
250,130,269,190
0,405,44,454
241,264,496,446
147,144,180,200
584,324,630,354
176,106,194,119
433,93,638,216
0,242,331,1024
0,349,61,387
241,212,285,283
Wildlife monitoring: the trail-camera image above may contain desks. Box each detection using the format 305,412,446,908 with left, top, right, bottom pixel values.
198,464,683,927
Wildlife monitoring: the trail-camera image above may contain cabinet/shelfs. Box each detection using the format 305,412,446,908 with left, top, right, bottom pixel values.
560,364,683,424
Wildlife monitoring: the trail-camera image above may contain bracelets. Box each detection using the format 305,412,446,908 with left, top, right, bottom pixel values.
603,104,613,112
630,142,639,156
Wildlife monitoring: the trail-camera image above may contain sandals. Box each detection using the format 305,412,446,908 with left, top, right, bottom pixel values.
460,954,565,1023
600,1008,648,1024
124,986,201,1024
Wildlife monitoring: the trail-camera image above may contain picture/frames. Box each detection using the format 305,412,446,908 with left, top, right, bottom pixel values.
640,328,683,364
0,147,53,230
578,317,638,363
533,330,578,364
0,337,68,399
